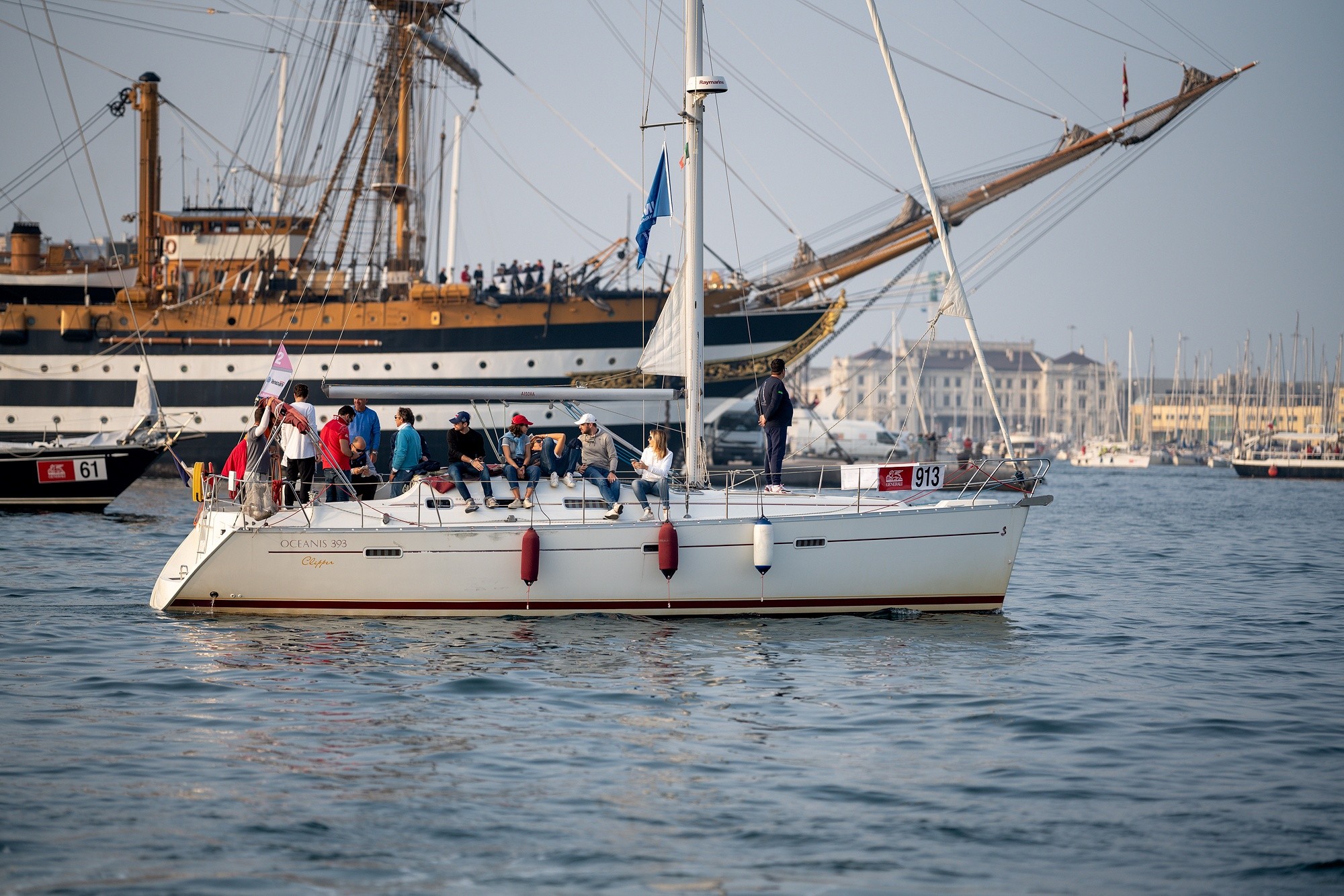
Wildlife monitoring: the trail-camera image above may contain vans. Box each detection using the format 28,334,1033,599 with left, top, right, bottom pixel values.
788,418,909,460
711,410,765,464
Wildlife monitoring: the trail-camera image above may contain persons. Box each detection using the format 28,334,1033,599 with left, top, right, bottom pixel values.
575,413,624,521
439,259,544,299
1081,441,1086,455
1307,442,1340,460
530,433,583,487
447,412,498,513
499,415,541,509
630,429,673,524
755,358,793,494
917,431,987,469
236,384,430,510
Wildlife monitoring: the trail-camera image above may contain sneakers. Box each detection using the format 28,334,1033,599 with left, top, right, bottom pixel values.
772,485,793,494
550,471,560,487
614,504,623,515
663,510,671,522
523,497,535,508
508,498,523,508
465,498,479,513
486,497,499,507
639,509,655,521
562,472,575,487
605,509,620,520
764,484,772,494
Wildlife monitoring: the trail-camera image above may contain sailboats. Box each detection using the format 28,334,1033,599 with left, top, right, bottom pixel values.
1230,312,1343,481
0,1,1262,618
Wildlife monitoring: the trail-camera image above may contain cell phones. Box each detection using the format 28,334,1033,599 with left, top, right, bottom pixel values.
630,459,638,462
360,466,368,470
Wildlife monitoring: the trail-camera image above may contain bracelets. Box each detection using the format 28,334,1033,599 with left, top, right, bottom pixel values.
522,465,527,471
316,453,322,455
516,467,520,471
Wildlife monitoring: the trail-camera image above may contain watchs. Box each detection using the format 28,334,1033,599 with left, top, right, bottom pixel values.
609,470,616,474
349,451,355,458
469,459,473,464
390,470,396,475
371,450,379,456
545,433,548,437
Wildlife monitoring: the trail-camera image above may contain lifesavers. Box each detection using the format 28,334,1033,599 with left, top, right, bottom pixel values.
165,239,177,254
829,448,843,458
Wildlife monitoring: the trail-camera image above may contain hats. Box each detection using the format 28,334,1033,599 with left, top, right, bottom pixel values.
449,412,470,424
574,414,596,425
512,415,534,425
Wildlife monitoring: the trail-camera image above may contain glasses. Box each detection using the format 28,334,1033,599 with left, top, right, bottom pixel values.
648,436,652,440
533,440,543,444
352,443,366,452
350,417,353,423
395,415,400,419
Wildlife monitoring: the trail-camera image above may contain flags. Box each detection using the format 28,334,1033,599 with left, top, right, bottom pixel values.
634,141,672,269
1268,422,1274,429
